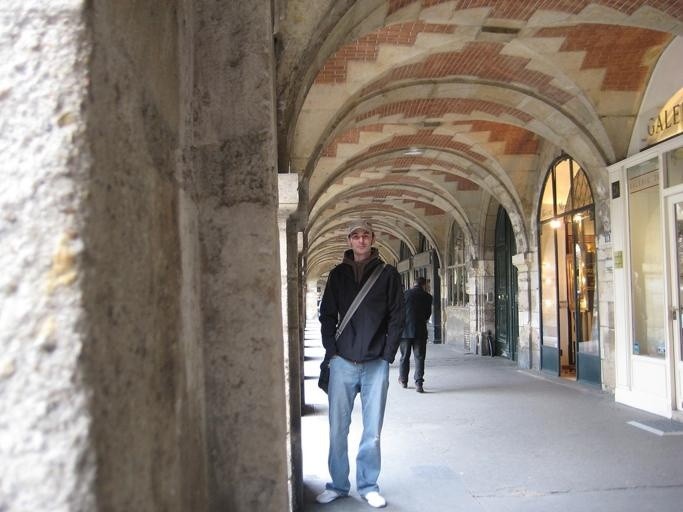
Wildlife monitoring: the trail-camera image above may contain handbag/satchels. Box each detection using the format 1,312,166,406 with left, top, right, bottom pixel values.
317,349,331,396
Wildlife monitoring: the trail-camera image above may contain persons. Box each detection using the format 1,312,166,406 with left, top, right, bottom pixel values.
398,276,433,392
314,218,406,508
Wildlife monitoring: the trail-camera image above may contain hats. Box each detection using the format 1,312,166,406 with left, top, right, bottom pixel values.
348,220,373,235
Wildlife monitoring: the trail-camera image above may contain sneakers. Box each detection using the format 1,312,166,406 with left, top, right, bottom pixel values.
414,383,423,392
316,489,350,504
360,490,387,508
398,376,408,388
